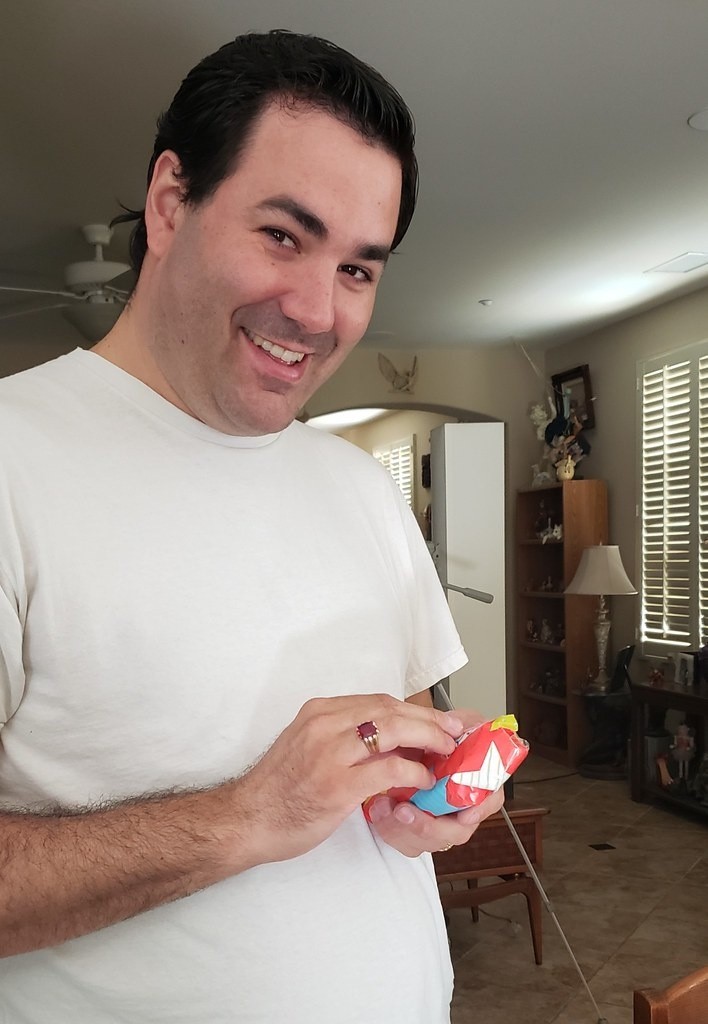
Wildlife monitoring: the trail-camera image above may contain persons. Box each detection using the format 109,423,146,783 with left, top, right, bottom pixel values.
0,29,504,1024
669,722,694,780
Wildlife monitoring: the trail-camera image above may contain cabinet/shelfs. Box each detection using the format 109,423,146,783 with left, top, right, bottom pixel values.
511,480,609,769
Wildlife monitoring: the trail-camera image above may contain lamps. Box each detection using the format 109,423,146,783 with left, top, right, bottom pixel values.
561,542,638,691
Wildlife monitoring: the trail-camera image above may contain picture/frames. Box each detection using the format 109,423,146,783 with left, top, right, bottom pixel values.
606,644,638,693
674,653,694,686
551,363,596,431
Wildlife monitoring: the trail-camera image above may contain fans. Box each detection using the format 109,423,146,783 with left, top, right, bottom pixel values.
1,224,131,321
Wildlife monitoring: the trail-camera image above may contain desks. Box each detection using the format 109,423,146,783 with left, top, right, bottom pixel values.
571,688,630,780
629,680,708,823
431,797,552,965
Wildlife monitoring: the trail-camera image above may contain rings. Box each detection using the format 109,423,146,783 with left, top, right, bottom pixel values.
356,720,378,756
439,843,452,852
